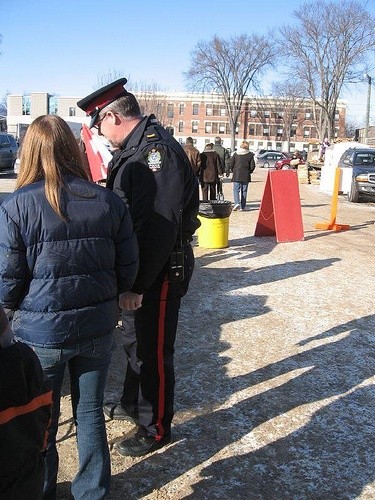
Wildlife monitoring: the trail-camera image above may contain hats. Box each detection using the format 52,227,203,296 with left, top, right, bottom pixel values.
77,78,127,130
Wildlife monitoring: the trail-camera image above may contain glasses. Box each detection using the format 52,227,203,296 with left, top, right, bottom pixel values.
93,111,118,129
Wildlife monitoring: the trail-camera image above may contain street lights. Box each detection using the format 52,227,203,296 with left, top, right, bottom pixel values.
364,73,372,145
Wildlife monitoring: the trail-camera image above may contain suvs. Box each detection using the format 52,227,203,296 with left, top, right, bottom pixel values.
337,148,375,202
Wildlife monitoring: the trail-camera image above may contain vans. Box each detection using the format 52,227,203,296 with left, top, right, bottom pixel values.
258,149,282,159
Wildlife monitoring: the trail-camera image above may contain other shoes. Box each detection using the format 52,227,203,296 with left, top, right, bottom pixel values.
240,209,244,211
233,204,240,211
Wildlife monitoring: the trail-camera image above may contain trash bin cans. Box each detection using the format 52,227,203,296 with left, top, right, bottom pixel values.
197,199,233,249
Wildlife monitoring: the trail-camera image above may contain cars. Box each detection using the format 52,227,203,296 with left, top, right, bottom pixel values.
256,152,282,168
275,152,306,170
13,158,20,179
0,133,19,170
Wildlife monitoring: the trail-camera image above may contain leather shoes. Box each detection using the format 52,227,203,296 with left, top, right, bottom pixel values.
103,402,137,424
117,433,172,457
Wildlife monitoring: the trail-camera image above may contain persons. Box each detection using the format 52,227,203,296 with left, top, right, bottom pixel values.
181,136,202,179
211,136,232,202
289,150,303,169
0,114,141,500
76,127,96,183
0,301,55,500
228,141,255,212
198,144,224,202
76,76,201,457
